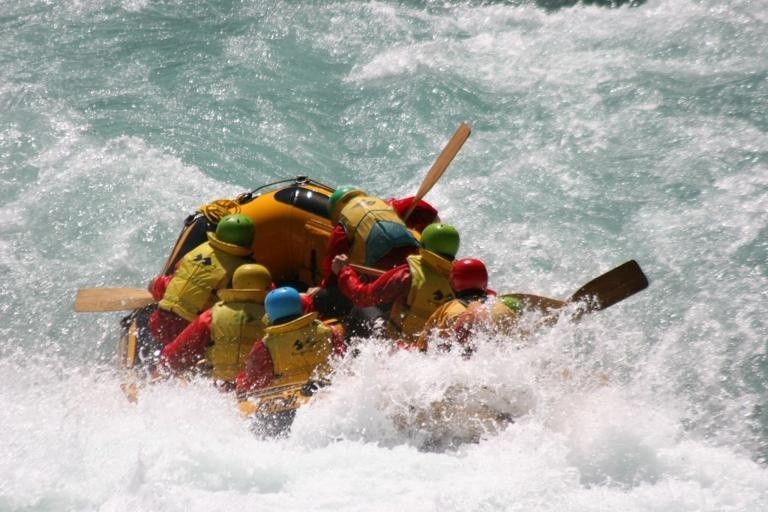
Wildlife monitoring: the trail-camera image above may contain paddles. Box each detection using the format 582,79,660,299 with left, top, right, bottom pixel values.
347,263,564,313
538,260,648,327
75,287,155,312
402,122,470,221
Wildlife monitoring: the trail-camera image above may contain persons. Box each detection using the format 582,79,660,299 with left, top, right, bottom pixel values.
330,223,461,348
230,287,349,439
315,186,421,320
148,213,256,347
159,262,324,405
413,258,533,354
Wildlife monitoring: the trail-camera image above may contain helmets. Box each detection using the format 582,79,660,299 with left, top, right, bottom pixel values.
499,296,524,314
450,257,488,295
326,186,357,214
231,264,272,289
264,287,302,321
420,222,460,256
215,215,254,247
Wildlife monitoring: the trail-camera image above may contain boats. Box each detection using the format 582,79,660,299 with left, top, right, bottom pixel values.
118,174,615,451
